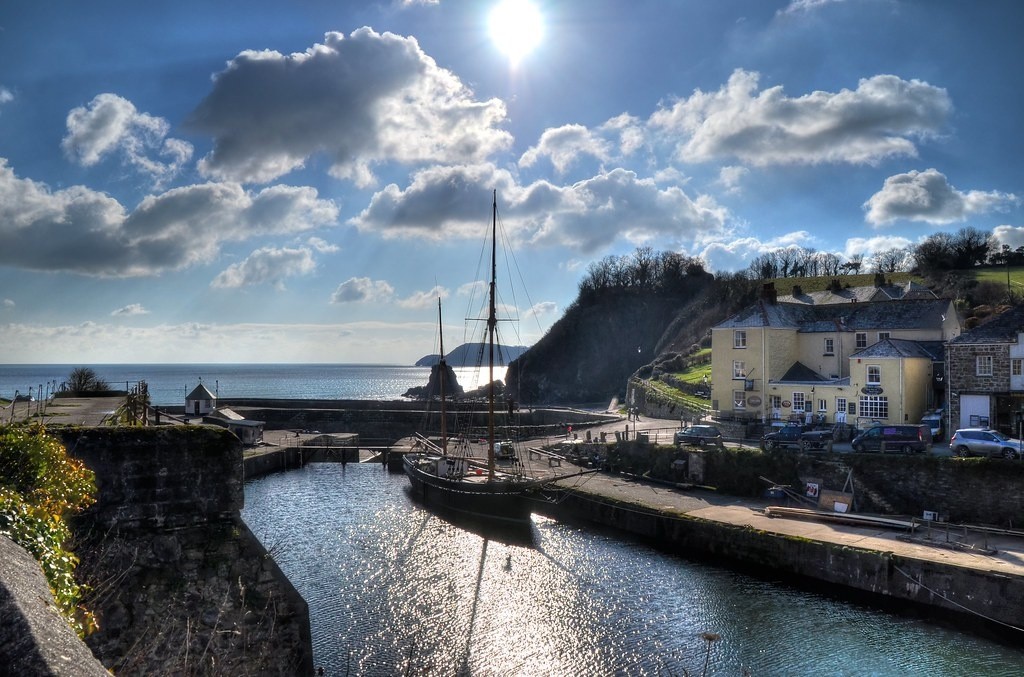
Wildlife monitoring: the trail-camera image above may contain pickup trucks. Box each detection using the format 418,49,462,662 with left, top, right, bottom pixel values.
764,425,833,450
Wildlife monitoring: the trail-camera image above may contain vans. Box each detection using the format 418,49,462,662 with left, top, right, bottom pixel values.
949,428,1024,459
851,425,933,455
921,414,945,442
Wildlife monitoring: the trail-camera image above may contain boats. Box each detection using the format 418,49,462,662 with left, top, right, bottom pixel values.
402,189,602,525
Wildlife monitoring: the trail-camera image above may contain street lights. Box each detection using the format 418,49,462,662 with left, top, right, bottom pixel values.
630,404,638,439
1016,411,1024,461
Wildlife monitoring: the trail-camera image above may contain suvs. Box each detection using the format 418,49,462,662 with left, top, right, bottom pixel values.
489,442,515,460
677,425,722,446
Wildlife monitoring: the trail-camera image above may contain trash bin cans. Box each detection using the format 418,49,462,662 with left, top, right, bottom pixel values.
637,431,649,442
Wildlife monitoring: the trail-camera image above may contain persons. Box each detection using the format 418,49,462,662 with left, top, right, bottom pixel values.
635,409,639,420
628,408,631,419
